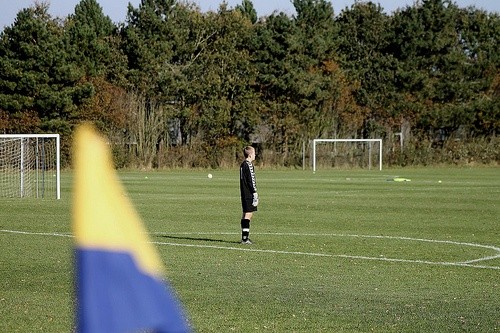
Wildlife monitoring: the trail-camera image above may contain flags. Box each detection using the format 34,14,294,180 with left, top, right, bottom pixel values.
71,123,189,333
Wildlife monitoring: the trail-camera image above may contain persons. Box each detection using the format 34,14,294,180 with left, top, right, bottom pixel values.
240,146,259,244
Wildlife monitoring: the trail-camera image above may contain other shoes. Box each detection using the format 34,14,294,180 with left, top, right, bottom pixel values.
241,240,251,244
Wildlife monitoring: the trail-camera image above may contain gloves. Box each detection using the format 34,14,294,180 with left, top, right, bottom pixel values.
252,193,258,206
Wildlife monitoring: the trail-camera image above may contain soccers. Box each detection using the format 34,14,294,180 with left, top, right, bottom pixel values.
207,172,213,179
